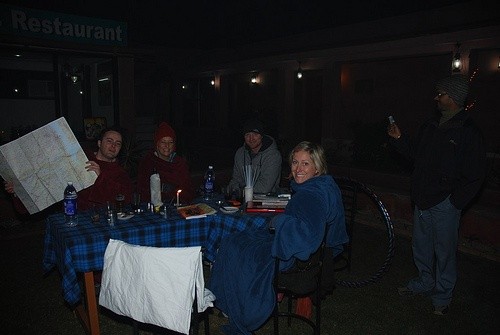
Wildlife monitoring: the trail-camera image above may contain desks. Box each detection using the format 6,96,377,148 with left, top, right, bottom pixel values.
42,188,286,335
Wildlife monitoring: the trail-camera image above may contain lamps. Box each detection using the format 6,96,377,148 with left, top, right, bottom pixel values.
451,45,463,73
247,72,258,88
72,72,78,84
296,62,303,81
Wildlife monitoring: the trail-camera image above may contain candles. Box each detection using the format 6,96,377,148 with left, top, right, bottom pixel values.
177,189,182,206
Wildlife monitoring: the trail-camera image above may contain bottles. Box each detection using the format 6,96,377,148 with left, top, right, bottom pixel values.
162,204,170,218
64,181,78,228
203,166,216,201
104,201,113,225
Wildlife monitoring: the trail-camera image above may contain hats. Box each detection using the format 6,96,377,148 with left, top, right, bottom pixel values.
155,124,176,142
436,74,478,107
242,118,265,135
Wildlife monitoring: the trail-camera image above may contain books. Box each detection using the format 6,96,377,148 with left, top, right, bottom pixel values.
178,204,207,220
245,196,288,212
197,203,217,216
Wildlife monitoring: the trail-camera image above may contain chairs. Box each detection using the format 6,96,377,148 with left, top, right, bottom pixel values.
98,238,217,335
253,228,325,335
324,179,357,272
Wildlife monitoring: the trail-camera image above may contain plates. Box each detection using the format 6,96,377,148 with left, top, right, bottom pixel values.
219,207,239,214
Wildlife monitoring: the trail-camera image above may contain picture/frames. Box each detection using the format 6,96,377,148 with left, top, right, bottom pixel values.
82,115,106,140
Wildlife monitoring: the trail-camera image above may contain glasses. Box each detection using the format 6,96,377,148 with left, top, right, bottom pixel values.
432,91,448,98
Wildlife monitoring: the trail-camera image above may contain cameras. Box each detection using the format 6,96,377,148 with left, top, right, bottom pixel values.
388,124,397,135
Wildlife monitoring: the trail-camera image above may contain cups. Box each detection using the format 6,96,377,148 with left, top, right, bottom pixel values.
130,193,140,210
114,192,126,214
89,205,100,225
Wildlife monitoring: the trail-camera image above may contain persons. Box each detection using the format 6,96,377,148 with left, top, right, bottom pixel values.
228,119,282,194
135,122,198,204
388,74,485,316
222,140,349,319
5,125,134,288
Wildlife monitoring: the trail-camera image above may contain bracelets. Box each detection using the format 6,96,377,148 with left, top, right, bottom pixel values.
397,136,400,138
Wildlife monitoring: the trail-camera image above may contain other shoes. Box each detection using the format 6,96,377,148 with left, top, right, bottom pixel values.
431,304,449,320
398,286,428,297
